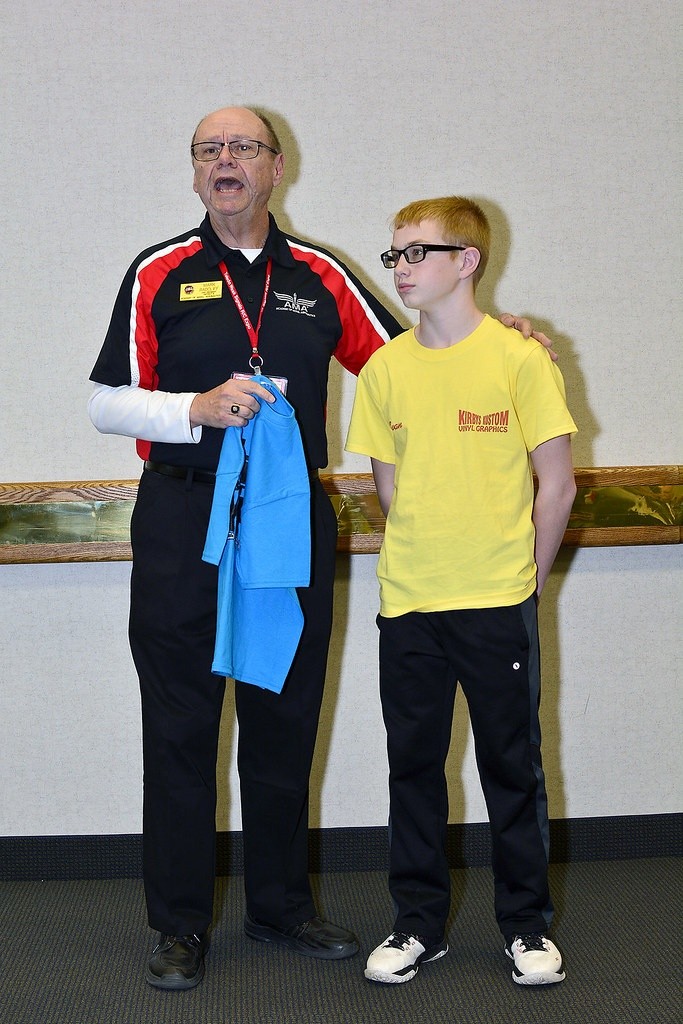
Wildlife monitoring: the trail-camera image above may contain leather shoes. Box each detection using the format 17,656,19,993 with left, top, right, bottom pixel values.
146,922,212,988
244,911,360,959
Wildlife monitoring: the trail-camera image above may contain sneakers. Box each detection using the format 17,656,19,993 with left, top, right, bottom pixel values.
365,931,450,983
504,933,566,984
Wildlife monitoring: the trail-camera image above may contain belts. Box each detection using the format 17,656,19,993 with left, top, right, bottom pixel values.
145,460,319,484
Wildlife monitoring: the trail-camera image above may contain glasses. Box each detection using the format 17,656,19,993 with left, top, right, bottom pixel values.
191,140,279,160
380,244,467,268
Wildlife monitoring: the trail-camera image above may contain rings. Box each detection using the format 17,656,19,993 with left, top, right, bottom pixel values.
230,405,240,414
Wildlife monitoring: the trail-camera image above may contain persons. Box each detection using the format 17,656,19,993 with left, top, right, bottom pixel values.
344,195,587,992
92,109,560,992
